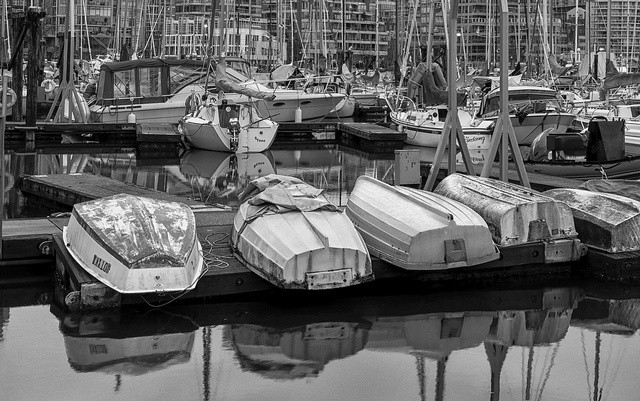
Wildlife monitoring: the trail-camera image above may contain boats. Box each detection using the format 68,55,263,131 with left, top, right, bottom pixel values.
433,174,589,267
60,337,194,382
489,307,573,348
478,88,574,145
345,176,501,270
390,104,495,155
180,91,280,154
227,175,371,290
389,308,496,360
570,298,640,336
451,134,640,184
540,187,640,253
179,150,275,185
63,193,203,293
229,322,370,377
81,57,344,134
